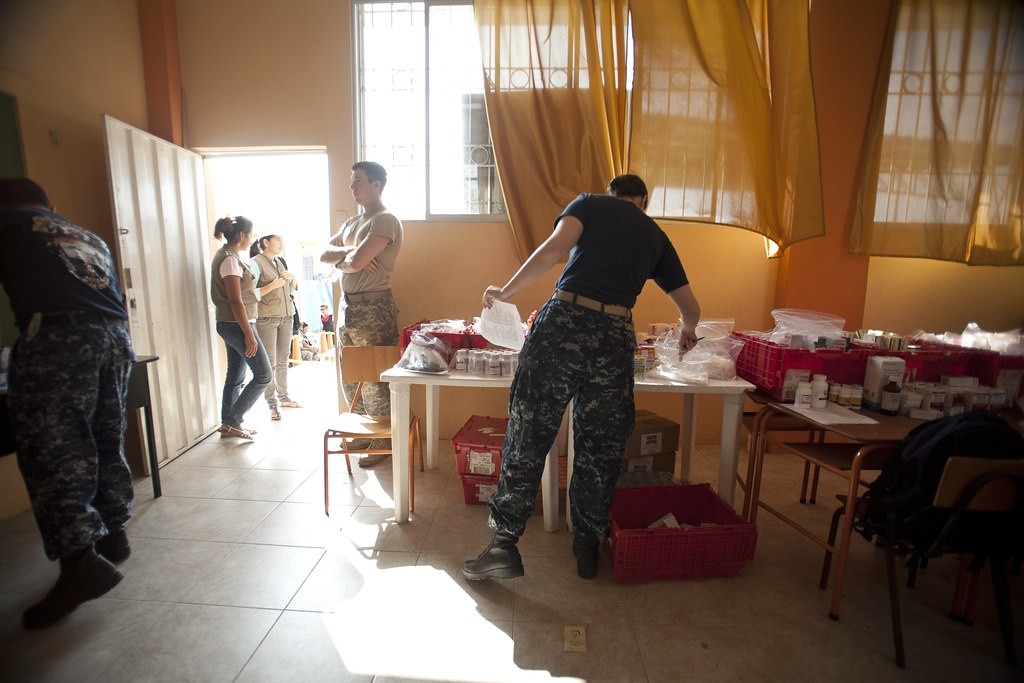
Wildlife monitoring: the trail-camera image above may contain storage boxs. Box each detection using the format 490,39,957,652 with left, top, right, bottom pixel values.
399,320,486,357
451,413,509,507
601,409,758,586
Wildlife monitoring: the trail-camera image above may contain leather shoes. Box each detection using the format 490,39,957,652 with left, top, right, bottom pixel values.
461,528,525,581
572,536,600,579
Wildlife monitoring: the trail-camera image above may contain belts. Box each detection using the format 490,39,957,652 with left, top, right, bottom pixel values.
551,288,631,317
39,312,127,328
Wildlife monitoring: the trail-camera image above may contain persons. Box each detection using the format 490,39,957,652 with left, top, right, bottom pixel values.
288,294,320,369
247,228,304,420
0,176,138,631
461,173,701,579
320,160,403,467
320,304,335,345
211,215,273,440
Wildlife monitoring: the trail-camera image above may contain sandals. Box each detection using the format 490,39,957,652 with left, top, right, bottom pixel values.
221,422,257,439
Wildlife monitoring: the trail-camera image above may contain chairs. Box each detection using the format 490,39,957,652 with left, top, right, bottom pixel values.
819,454,1024,671
323,346,425,514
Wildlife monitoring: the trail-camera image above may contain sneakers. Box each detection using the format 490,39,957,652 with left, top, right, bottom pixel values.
340,437,371,451
359,438,392,466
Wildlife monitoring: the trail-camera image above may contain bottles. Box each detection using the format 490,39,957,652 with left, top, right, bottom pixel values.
455,346,520,377
794,373,864,410
879,375,901,416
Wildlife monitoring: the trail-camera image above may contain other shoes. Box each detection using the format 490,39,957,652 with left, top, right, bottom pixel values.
22,545,124,632
98,530,130,560
271,408,280,420
280,398,302,408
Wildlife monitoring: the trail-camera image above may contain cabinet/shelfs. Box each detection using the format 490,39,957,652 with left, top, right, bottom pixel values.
731,331,1024,420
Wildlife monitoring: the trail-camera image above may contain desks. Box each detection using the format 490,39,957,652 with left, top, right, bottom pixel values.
738,389,928,621
380,363,756,533
0,356,163,499
